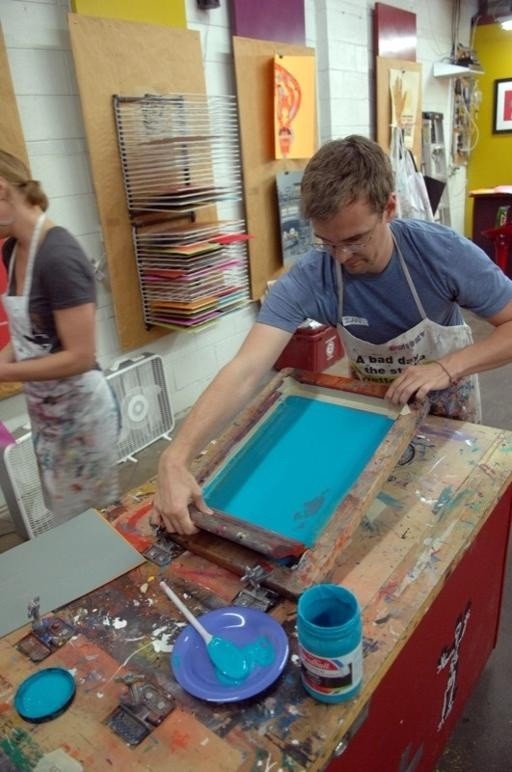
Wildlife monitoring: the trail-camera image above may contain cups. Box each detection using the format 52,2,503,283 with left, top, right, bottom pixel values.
296,582,364,705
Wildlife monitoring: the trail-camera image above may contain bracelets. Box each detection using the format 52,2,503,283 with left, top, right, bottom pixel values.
424,358,459,388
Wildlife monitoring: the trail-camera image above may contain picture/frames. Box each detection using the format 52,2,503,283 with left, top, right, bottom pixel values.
493,78,512,134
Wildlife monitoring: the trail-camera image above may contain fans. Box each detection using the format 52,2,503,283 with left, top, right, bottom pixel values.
1,351,176,538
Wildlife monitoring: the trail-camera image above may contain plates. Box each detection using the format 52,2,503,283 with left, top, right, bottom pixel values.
169,606,291,705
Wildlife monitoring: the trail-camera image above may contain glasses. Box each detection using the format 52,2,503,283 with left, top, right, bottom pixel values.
311,212,379,252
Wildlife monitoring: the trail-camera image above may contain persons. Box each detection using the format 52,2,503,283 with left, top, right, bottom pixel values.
147,131,510,539
0,147,125,518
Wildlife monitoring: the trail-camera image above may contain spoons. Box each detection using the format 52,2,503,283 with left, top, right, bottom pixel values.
159,577,247,678
